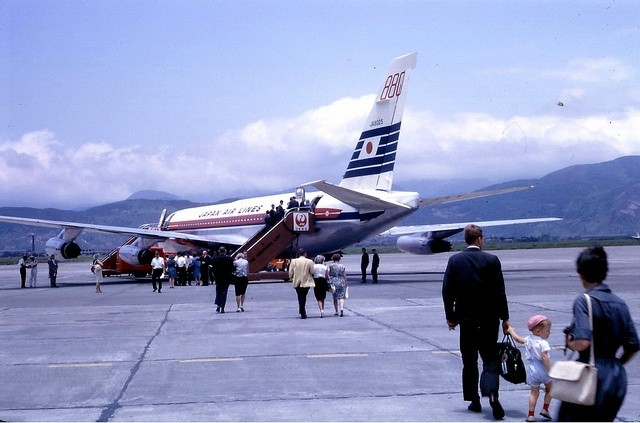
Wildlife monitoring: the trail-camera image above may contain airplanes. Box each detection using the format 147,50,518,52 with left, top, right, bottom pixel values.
0,52,563,280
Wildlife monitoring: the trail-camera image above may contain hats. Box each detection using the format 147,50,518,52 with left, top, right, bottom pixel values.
527,315,548,331
155,251,159,254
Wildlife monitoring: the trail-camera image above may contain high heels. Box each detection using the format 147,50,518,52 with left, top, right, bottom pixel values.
319,310,324,318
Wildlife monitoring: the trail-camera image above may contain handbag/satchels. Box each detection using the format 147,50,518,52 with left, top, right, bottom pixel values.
327,282,336,293
91,266,94,273
548,360,598,405
345,286,349,298
95,264,101,270
497,333,526,383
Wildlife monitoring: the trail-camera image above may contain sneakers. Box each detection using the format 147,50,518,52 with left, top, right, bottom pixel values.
172,286,174,288
240,306,244,311
526,414,534,422
334,311,338,315
221,309,225,312
216,307,220,311
170,285,172,288
153,289,157,292
198,283,200,286
340,309,343,317
539,409,552,420
158,289,161,293
236,308,240,312
195,282,198,286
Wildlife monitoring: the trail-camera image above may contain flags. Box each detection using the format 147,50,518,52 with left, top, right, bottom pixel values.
356,135,382,161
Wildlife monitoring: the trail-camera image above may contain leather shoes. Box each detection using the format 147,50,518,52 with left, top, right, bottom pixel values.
468,403,481,412
301,315,307,319
489,392,505,419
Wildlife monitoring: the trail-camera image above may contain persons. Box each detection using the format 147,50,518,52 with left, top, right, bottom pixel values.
312,254,330,318
441,223,511,420
199,250,210,285
264,210,272,228
269,203,275,217
167,255,177,288
47,253,59,287
173,255,178,286
279,199,285,217
329,253,348,316
274,207,281,221
92,252,104,293
165,254,169,272
506,314,552,421
17,254,28,288
288,248,317,319
150,250,165,293
209,246,236,313
232,251,250,312
292,196,299,206
183,251,193,285
360,247,369,283
27,255,38,288
210,249,218,284
371,248,379,283
299,198,306,211
557,243,640,421
176,251,188,285
266,257,291,282
189,255,202,285
287,196,293,208
